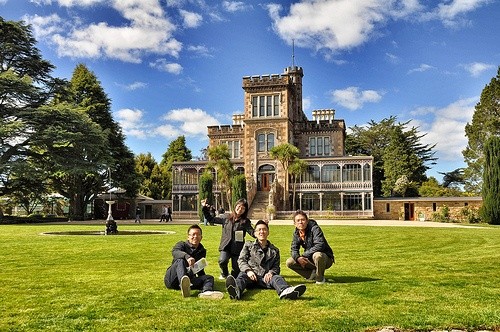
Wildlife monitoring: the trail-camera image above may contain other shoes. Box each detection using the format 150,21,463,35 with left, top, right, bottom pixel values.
198,291,224,300
180,275,191,298
316,278,325,285
308,270,315,280
219,275,225,280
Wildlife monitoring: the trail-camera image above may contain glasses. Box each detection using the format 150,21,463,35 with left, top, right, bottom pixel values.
188,233,201,236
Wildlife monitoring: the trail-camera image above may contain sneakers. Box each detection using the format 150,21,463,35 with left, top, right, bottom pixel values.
280,284,306,300
226,275,240,300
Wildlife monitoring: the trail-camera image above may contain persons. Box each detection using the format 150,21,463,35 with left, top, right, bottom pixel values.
165,224,224,300
226,220,306,301
201,198,225,226
286,210,335,285
200,198,269,280
135,207,142,223
159,205,172,222
